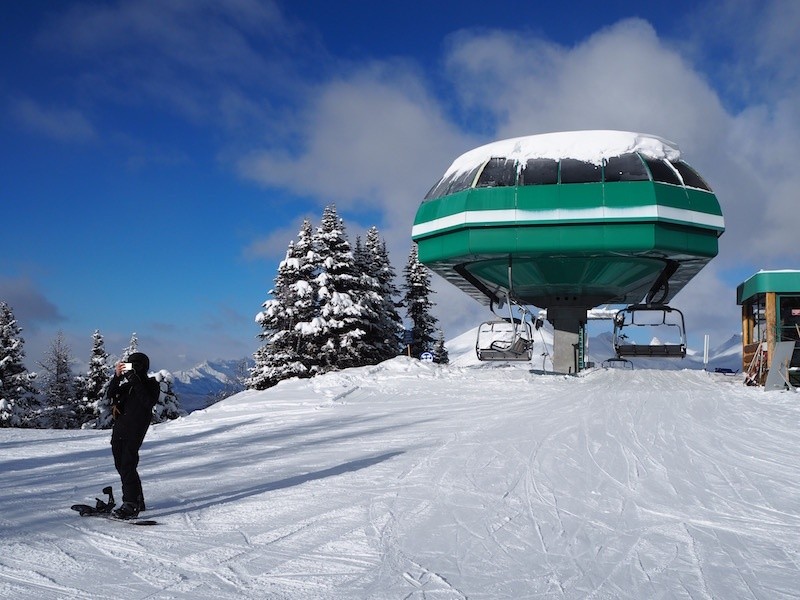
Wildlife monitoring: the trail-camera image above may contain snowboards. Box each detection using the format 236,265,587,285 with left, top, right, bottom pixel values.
72,499,157,528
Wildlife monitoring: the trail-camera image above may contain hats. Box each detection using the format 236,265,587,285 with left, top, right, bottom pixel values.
124,353,150,368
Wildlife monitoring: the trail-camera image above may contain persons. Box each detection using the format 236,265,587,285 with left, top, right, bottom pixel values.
106,352,159,520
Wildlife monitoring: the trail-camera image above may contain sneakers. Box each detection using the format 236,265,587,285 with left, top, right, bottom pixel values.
112,503,140,519
140,500,145,510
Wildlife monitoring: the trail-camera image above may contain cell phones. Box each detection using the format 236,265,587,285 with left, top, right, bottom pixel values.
121,363,132,370
